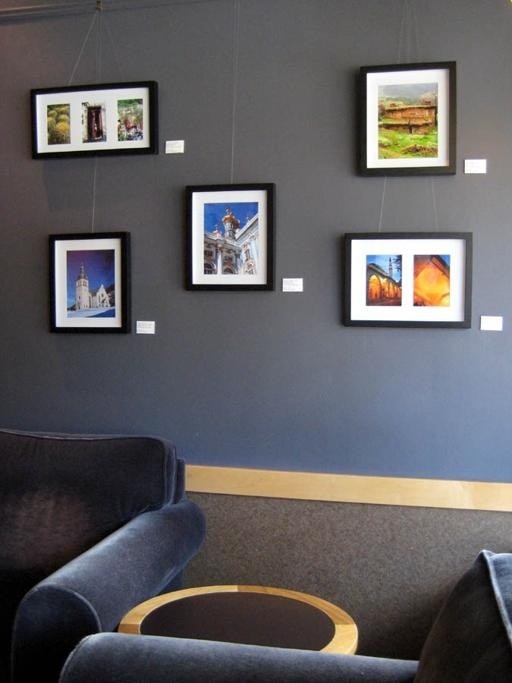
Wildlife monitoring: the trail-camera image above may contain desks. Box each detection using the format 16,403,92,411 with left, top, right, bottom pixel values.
117,583,359,657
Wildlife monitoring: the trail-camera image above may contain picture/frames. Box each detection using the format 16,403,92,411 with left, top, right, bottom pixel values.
359,60,458,175
343,232,473,329
49,232,131,334
30,80,160,160
185,183,276,291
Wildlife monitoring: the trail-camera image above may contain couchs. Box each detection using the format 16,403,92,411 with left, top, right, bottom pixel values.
0,428,208,683
60,552,512,683
